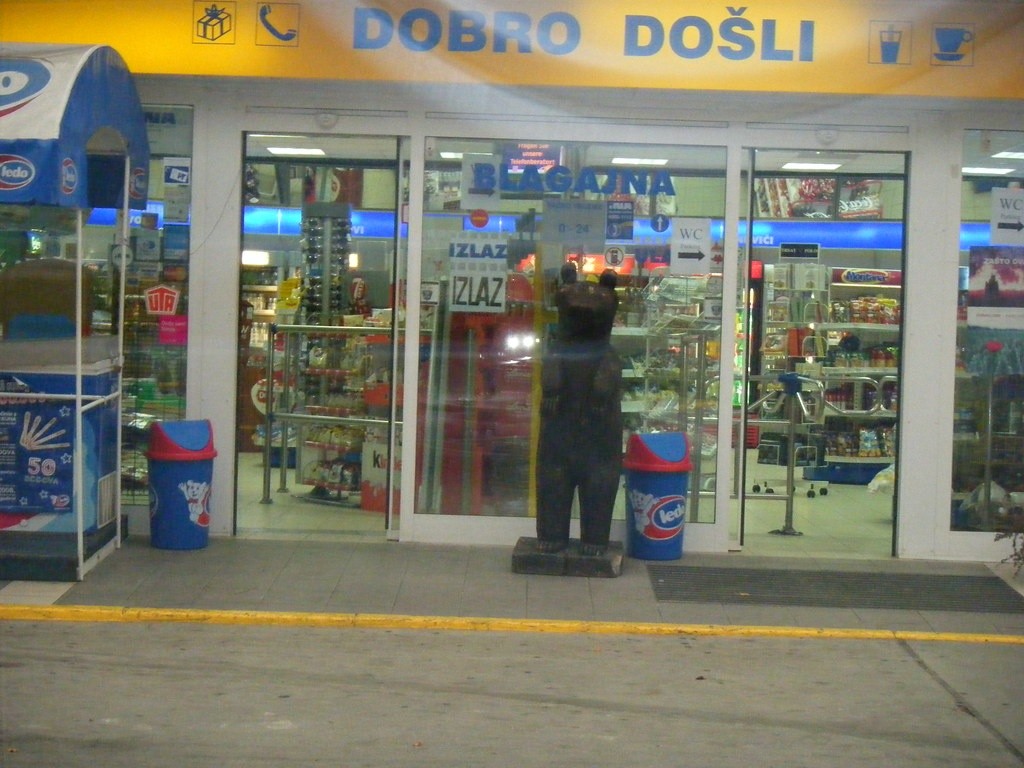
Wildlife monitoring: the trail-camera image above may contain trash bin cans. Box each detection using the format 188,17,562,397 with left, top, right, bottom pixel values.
620,431,694,561
141,419,219,551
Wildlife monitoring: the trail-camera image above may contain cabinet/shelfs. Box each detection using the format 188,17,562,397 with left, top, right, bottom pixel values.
240,283,281,317
609,281,901,456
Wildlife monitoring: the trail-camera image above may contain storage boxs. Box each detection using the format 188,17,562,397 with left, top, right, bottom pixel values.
824,456,894,484
0,260,92,342
